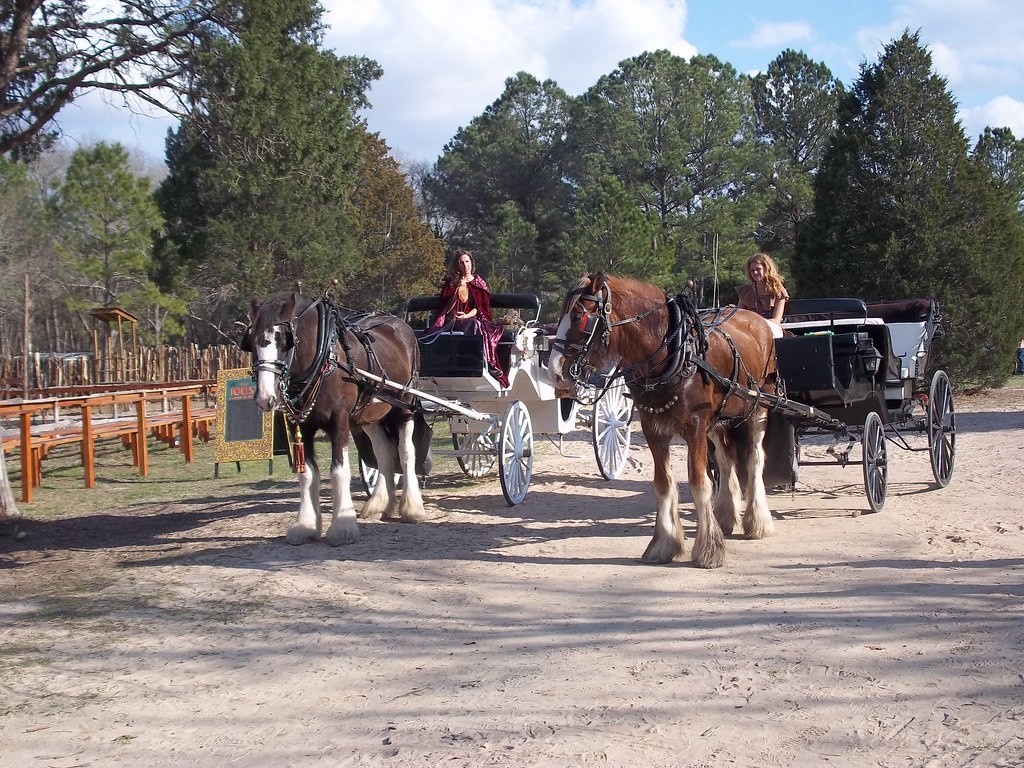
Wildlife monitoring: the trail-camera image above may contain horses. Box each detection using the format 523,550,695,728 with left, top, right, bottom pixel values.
240,291,428,547
546,270,777,569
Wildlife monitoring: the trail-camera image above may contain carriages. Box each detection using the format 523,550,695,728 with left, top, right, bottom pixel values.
239,279,631,546
548,270,956,568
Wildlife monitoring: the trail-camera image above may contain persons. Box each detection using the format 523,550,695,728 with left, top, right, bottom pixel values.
738,254,789,323
1017,340,1024,372
431,249,503,379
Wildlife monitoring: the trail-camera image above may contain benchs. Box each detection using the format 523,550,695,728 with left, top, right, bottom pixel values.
1,407,218,488
781,298,929,385
404,294,558,361
